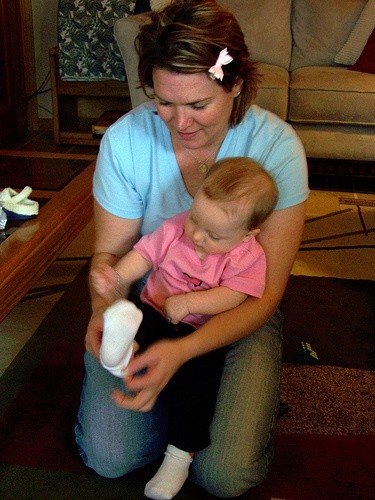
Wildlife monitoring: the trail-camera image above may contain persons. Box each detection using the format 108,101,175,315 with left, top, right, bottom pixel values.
76,1,309,497
90,155,281,500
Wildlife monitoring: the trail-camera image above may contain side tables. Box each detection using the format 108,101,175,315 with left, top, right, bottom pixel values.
49,46,131,146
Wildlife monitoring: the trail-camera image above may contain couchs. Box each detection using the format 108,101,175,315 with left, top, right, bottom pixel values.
114,0,375,162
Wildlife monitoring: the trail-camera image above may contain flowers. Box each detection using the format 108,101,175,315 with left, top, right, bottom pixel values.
208,48,233,81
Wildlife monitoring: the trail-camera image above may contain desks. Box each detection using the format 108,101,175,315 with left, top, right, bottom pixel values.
0,149,96,325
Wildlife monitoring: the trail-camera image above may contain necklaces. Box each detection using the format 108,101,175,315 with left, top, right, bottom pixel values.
198,130,218,174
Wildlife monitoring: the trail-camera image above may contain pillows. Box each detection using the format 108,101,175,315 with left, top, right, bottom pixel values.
335,0,375,74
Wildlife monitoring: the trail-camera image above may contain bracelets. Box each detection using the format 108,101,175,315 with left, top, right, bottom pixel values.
114,268,120,288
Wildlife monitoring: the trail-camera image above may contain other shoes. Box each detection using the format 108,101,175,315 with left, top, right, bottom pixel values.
0,186,39,223
